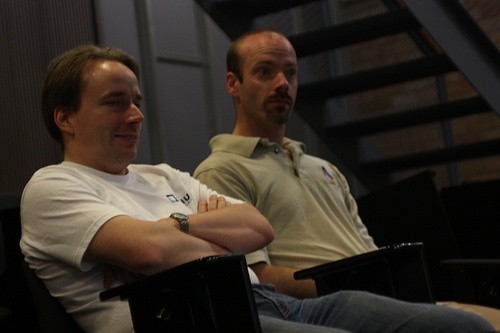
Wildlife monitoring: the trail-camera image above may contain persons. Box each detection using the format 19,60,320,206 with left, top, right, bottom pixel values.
192,31,500,331
19,44,496,333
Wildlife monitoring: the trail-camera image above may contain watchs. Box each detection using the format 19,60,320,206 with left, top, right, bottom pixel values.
169,212,189,232
